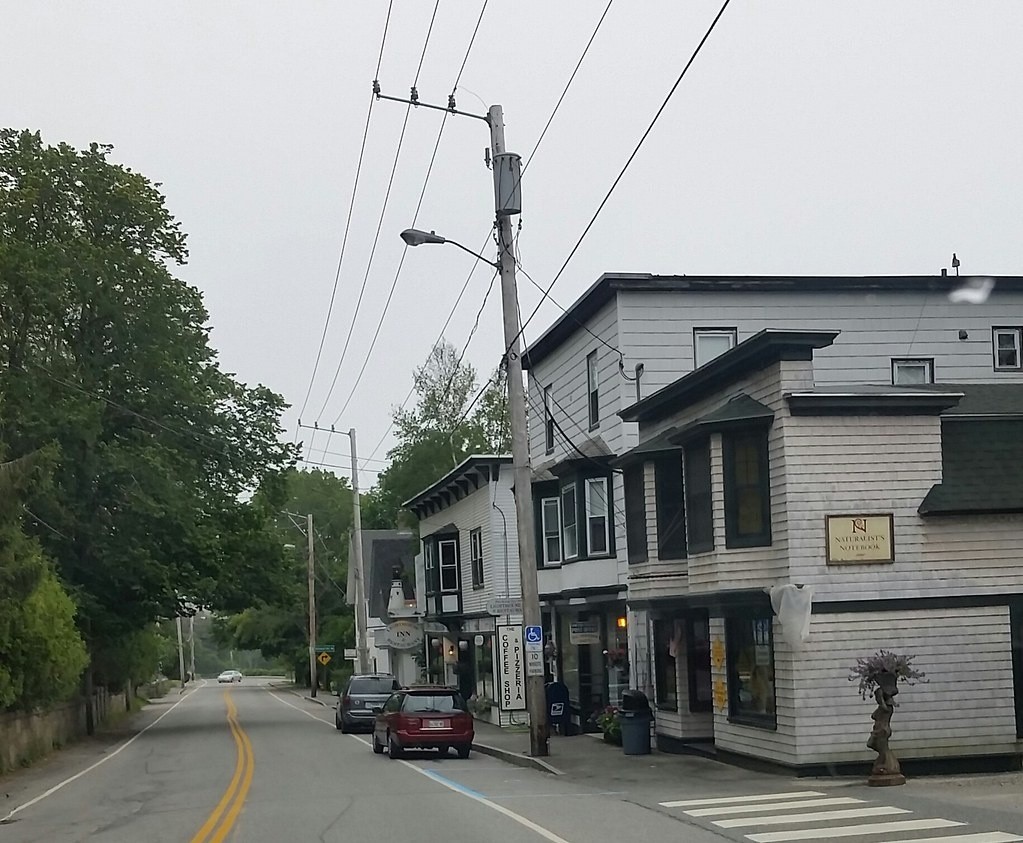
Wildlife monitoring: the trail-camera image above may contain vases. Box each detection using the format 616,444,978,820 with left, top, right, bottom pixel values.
603,729,623,747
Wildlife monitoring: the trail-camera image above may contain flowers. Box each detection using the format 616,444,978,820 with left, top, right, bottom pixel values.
595,706,623,736
603,648,630,670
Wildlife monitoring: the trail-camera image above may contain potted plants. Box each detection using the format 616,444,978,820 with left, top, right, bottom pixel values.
467,693,494,717
848,649,930,786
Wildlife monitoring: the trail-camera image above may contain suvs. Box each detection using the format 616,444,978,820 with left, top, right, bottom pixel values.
334,672,405,734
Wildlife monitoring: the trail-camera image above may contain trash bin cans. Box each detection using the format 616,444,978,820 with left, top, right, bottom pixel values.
616,690,651,755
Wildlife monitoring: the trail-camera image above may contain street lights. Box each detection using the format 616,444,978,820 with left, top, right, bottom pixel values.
284,543,317,699
399,229,552,757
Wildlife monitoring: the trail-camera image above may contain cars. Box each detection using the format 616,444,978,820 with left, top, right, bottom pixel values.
371,685,476,759
217,670,243,682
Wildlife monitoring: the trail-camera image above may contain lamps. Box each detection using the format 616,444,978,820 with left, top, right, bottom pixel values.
449,645,454,655
